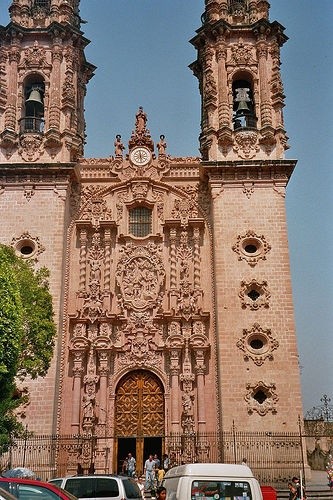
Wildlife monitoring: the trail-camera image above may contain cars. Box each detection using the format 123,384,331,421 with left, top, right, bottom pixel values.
47,475,143,500
0,475,78,500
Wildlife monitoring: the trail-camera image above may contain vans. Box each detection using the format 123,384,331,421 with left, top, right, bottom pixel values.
160,462,264,500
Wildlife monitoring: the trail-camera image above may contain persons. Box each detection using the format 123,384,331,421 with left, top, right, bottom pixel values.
144,455,158,490
182,388,194,423
288,477,306,500
154,454,172,487
156,487,166,500
82,384,96,417
241,458,247,465
114,135,125,155
157,134,167,154
126,453,136,477
122,457,128,476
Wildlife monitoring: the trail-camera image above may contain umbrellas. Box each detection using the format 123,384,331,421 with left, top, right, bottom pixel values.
2,467,35,479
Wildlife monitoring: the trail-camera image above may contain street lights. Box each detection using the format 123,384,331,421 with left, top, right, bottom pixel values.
7,410,27,470
